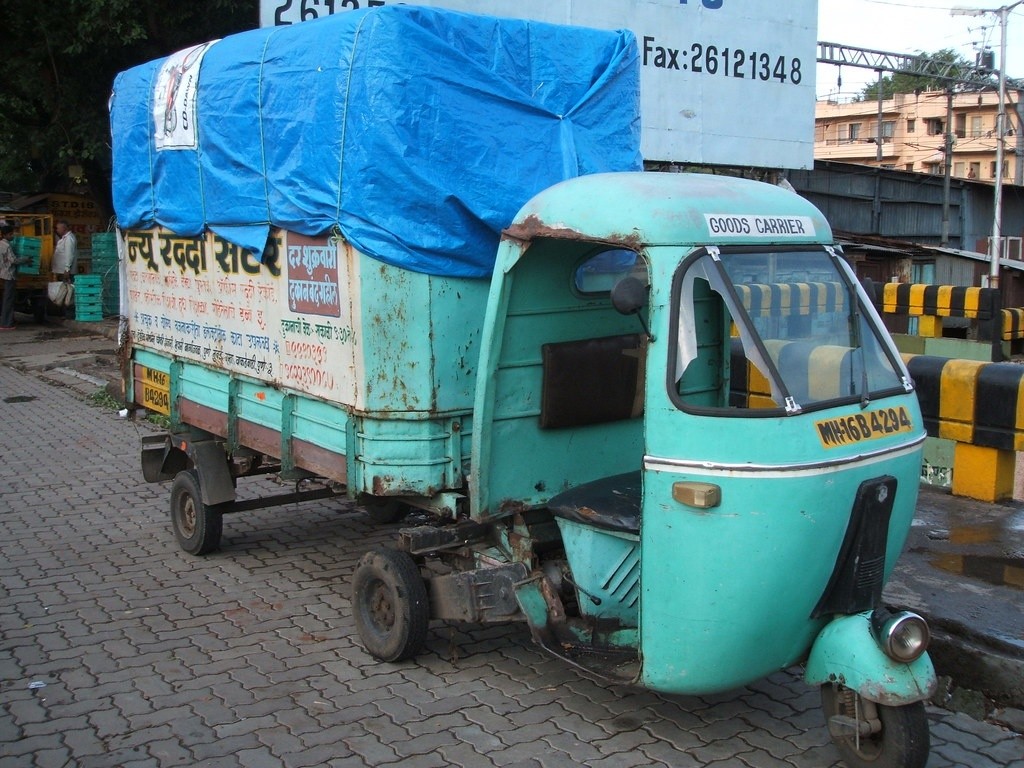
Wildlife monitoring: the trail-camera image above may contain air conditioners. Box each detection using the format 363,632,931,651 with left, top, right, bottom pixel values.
987,236,1021,261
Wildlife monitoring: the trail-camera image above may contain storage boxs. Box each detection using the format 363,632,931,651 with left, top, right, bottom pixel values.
9,234,43,247
11,245,41,257
17,266,40,274
73,233,120,321
16,255,41,266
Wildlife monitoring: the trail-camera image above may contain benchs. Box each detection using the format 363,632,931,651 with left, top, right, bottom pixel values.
546,471,642,535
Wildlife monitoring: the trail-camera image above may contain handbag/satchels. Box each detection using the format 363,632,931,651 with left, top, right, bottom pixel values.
64,284,75,306
47,281,68,306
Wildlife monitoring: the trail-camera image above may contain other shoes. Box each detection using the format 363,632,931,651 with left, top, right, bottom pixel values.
0,324,16,330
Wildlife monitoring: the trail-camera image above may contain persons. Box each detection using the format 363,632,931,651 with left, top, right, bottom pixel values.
50,221,78,318
0,225,34,331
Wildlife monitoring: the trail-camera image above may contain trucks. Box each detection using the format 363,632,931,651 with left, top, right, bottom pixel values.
115,4,939,767
